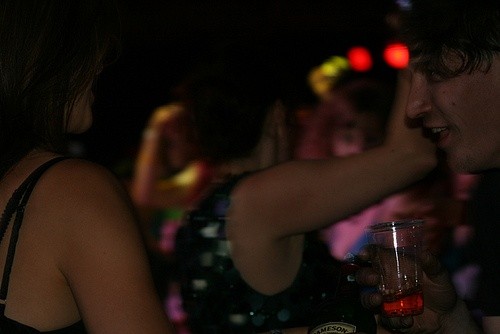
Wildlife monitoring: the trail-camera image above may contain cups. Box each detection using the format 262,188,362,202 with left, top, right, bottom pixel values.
367,219,425,315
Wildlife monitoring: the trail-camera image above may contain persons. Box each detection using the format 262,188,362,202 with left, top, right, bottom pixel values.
171,36,422,334
132,91,209,334
0,1,171,334
406,0,500,176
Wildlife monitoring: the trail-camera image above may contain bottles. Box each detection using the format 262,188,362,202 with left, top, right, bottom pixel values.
306,263,378,334
